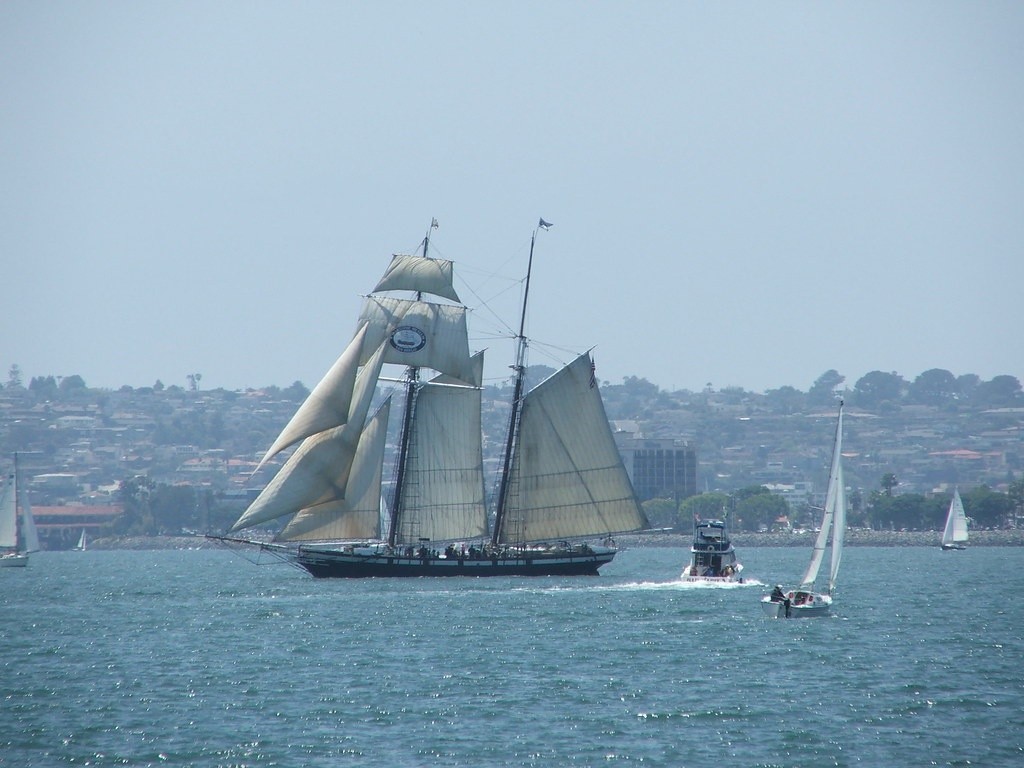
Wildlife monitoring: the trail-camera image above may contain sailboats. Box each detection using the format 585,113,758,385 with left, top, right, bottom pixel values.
72,527,88,552
759,399,847,618
941,484,969,551
201,215,655,580
0,449,42,569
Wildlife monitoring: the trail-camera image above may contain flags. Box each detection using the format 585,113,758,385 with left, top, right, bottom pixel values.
538,218,553,232
432,218,439,229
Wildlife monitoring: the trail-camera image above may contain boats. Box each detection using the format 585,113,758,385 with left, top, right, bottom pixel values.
682,502,744,587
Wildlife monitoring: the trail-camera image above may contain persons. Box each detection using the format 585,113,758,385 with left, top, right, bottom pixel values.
445,544,458,557
404,544,440,558
460,543,465,555
468,545,496,558
690,567,698,576
723,564,733,576
580,542,588,553
771,585,785,602
706,565,713,576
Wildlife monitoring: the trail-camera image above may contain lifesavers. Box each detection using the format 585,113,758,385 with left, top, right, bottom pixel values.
707,546,714,551
